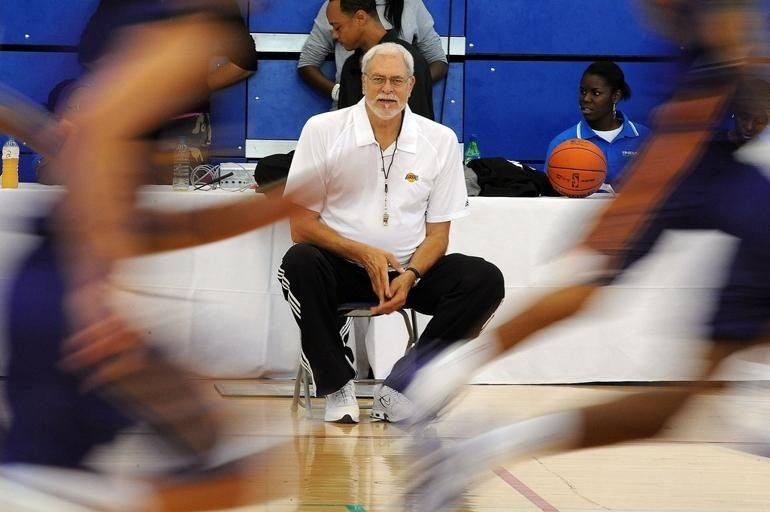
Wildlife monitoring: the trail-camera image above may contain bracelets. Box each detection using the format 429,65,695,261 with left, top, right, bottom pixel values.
405,267,421,288
331,83,340,102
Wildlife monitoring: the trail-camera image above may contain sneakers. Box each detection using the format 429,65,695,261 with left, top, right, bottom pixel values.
323,379,360,425
369,383,415,423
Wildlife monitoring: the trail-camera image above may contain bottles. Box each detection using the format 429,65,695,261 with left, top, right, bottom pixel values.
173,135,190,191
2,135,19,189
464,136,480,164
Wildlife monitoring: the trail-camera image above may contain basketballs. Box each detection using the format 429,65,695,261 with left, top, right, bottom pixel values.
547,139,606,198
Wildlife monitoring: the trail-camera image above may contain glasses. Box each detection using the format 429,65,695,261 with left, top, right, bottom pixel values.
363,72,412,86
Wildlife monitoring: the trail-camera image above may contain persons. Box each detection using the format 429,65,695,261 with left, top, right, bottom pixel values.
398,0,770,512
543,60,653,193
281,42,506,424
297,0,448,112
78,1,258,157
0,0,376,512
33,76,212,185
326,0,435,122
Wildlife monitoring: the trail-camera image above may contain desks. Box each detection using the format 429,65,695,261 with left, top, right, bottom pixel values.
0,184,732,384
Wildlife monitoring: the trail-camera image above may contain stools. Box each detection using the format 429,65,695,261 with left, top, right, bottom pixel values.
290,305,422,419
